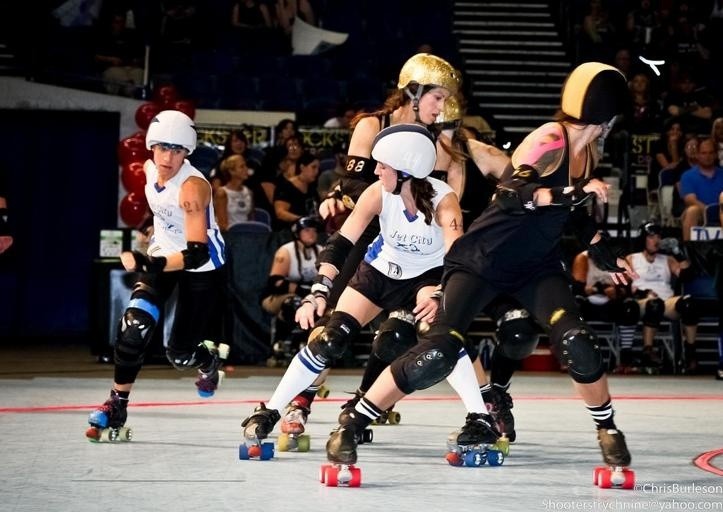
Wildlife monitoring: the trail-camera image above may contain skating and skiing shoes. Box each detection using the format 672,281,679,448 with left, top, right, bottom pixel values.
592,427,634,490
239,401,281,459
320,387,401,487
86,389,133,444
610,347,701,374
446,391,516,467
278,396,310,452
266,339,296,367
193,340,229,399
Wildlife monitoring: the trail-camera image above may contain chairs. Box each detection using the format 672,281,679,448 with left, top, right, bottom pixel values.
186,146,723,373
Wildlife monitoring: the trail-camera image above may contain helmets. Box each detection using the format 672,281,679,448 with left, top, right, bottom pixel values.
639,223,660,244
398,53,463,132
372,123,436,179
292,216,317,232
145,111,197,155
563,62,627,126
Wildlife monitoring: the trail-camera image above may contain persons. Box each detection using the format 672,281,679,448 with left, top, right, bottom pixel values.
95,0,723,382
327,60,641,468
238,49,539,449
87,110,220,427
